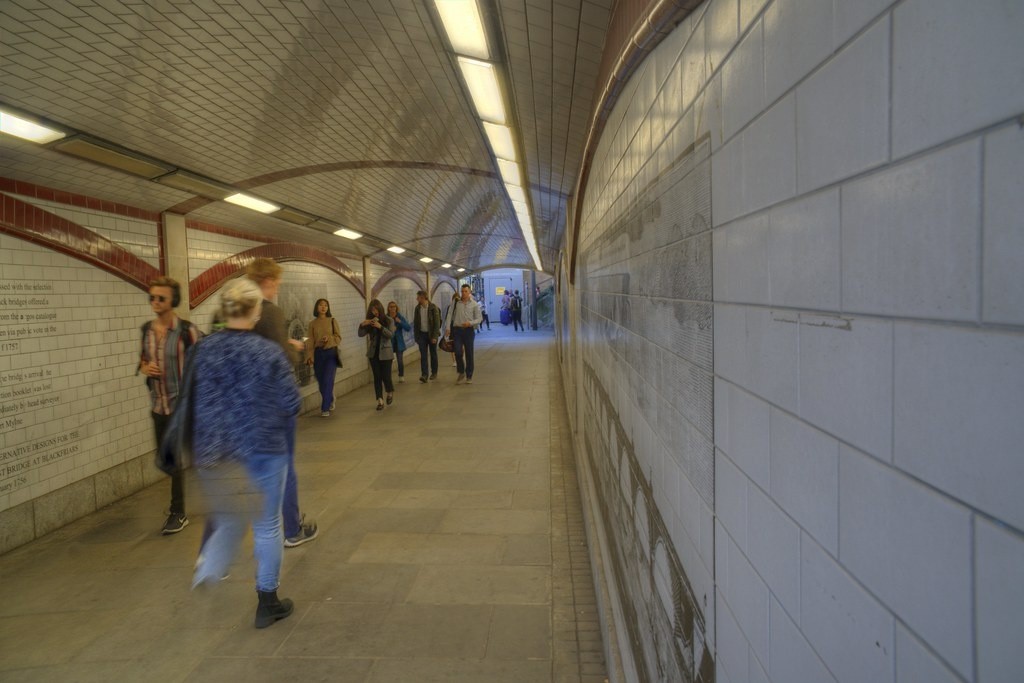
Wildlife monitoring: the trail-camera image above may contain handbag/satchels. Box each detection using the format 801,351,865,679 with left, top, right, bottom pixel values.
336,348,343,368
439,336,455,352
151,341,204,476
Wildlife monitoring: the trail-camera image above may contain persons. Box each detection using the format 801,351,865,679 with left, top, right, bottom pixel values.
386,302,411,383
445,284,483,386
536,287,540,295
500,289,524,331
181,277,303,627
476,296,491,333
414,290,440,383
307,298,342,418
445,293,465,366
357,299,395,411
193,256,320,581
138,276,205,536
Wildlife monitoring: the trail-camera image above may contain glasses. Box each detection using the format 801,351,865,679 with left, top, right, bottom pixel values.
150,294,165,302
389,306,396,308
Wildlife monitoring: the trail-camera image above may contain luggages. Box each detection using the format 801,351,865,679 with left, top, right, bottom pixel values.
500,308,511,324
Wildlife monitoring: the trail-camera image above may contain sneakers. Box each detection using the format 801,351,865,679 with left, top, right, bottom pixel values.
161,512,189,535
284,523,318,548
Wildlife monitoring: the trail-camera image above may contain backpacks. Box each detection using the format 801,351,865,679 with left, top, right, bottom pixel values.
514,296,522,309
416,304,443,329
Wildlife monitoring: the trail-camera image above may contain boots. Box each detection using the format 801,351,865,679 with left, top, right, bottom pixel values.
255,591,294,628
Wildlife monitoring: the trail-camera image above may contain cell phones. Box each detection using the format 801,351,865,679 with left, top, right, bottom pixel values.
299,337,308,342
372,317,379,323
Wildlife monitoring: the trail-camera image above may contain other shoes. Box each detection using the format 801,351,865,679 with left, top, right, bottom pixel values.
386,391,394,405
451,361,456,367
487,329,491,330
466,378,472,384
376,403,384,410
456,373,465,384
329,395,336,410
477,329,480,333
321,409,329,416
481,329,483,331
419,376,428,383
429,374,437,380
399,376,404,383
